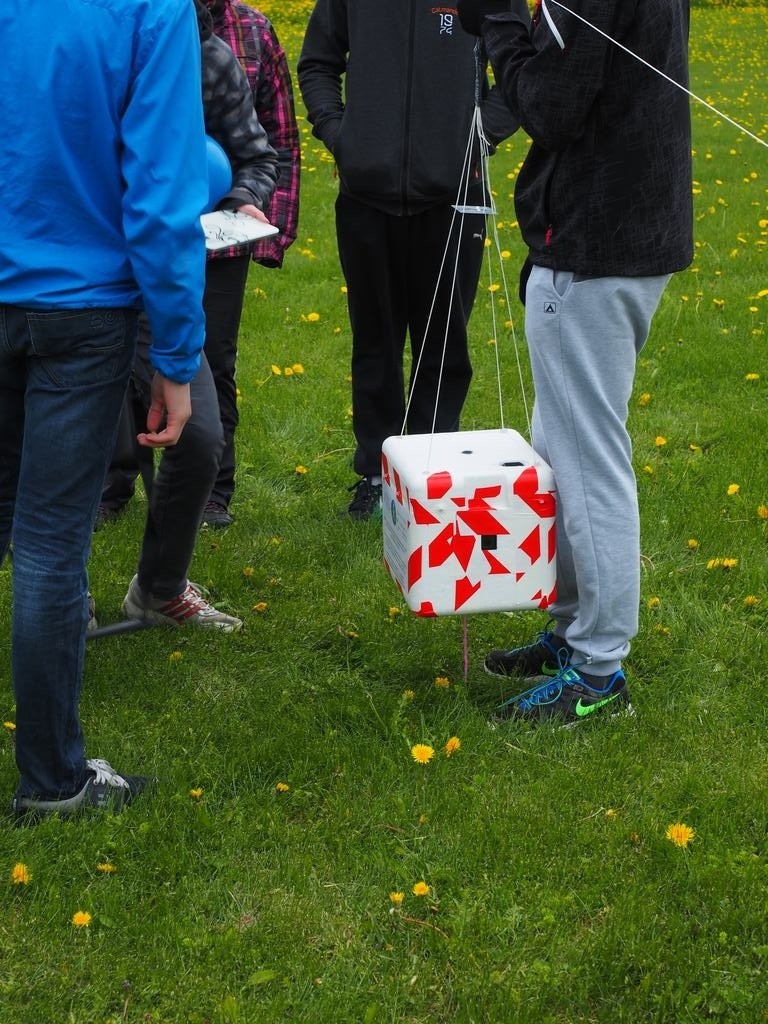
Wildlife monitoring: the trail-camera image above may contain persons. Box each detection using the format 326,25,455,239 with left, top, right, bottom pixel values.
290,1,530,517
1,0,216,822
70,0,305,534
109,0,277,641
449,0,689,727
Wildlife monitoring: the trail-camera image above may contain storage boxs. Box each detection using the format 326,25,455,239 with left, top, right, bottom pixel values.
383,428,559,618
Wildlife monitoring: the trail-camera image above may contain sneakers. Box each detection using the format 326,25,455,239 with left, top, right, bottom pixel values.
495,665,637,736
12,758,148,828
483,630,574,682
202,501,232,529
91,505,118,535
120,573,243,634
347,475,382,521
